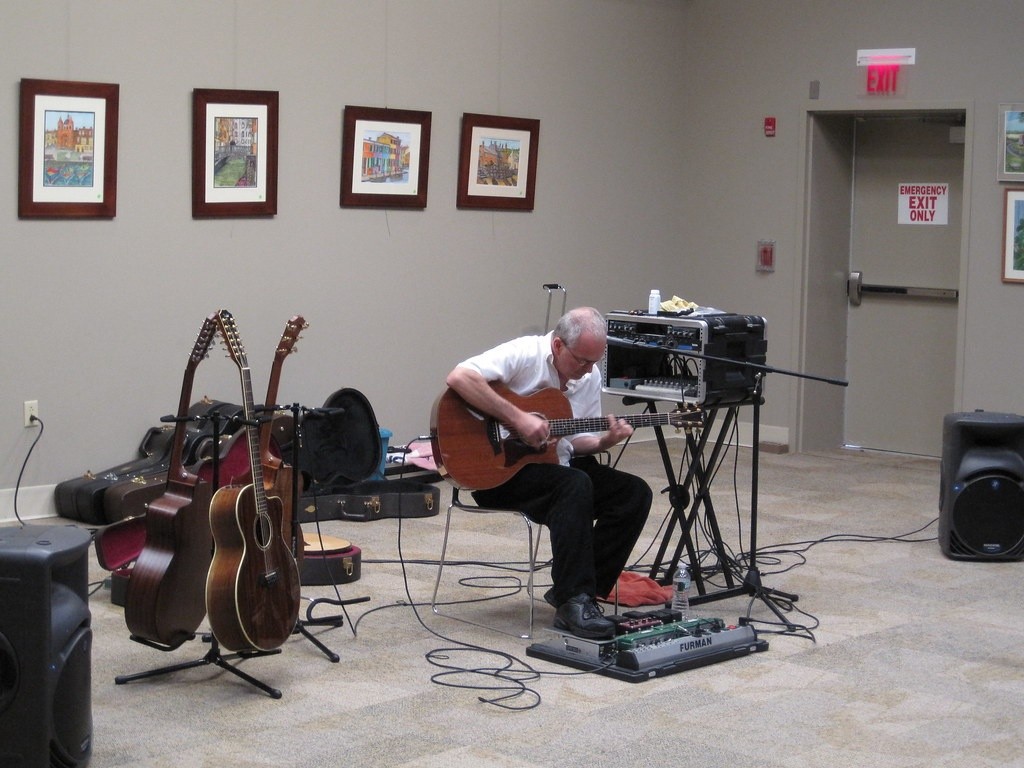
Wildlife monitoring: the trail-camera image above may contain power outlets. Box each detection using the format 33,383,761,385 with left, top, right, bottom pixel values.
24,399,39,426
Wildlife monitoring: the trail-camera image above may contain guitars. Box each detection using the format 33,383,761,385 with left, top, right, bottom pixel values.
116,316,218,652
204,307,302,652
242,314,312,553
428,378,710,492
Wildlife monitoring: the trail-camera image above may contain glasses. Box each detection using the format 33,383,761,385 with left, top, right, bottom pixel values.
558,338,596,367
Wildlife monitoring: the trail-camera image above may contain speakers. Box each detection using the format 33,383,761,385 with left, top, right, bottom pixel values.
939,409,1024,562
0,525,93,768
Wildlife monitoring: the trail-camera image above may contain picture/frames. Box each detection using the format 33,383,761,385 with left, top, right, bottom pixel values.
17,78,119,218
1001,187,1024,283
339,105,432,207
457,112,539,209
996,103,1023,182
193,88,280,216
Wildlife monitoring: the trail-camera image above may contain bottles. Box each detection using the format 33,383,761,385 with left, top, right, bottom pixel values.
671,563,690,621
648,290,661,314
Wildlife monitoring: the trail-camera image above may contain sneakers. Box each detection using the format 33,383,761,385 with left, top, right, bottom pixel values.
555,593,616,638
544,587,604,616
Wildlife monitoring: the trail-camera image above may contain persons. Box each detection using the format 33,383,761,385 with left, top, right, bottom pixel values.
446,307,652,639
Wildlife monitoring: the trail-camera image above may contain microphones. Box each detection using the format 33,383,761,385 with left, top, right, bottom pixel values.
605,335,645,350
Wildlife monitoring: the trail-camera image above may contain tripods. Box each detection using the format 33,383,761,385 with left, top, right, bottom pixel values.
115,404,344,699
624,340,849,633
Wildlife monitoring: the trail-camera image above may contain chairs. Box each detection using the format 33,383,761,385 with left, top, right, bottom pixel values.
432,489,553,638
